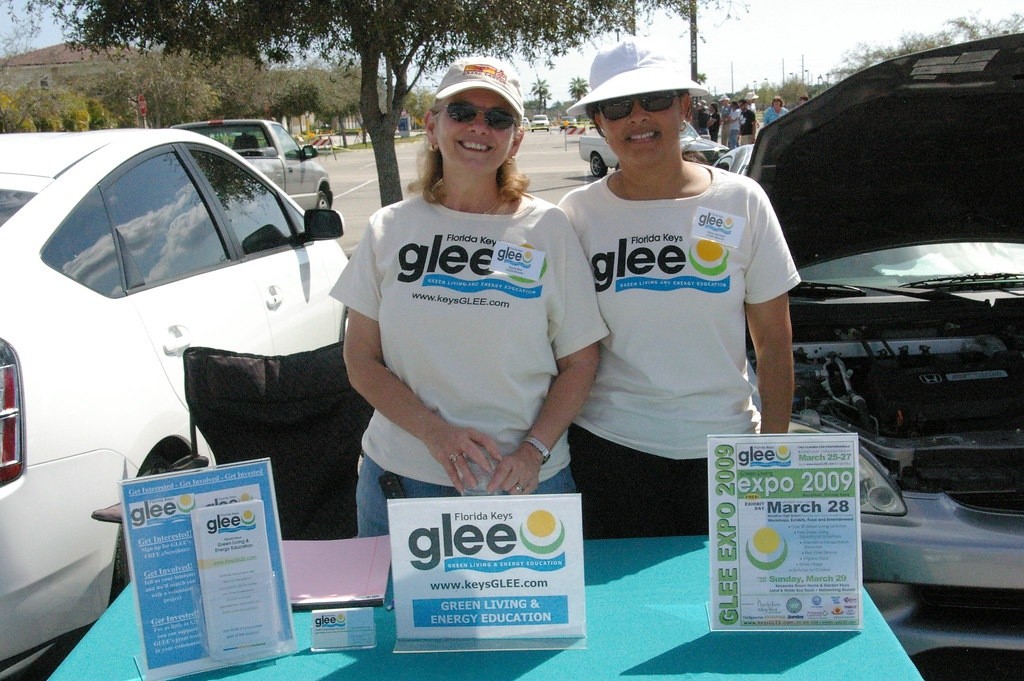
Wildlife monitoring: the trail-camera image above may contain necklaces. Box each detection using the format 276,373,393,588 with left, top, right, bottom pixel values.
483,191,500,213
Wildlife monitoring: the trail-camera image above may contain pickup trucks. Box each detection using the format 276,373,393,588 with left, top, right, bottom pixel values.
171,119,334,213
579,120,729,180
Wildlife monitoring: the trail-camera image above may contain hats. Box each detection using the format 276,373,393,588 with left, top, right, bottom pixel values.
436,57,525,122
718,95,731,101
698,100,708,109
745,92,759,99
566,38,709,115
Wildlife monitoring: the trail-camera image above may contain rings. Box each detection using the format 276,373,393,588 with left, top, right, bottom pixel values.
450,453,463,463
515,484,522,491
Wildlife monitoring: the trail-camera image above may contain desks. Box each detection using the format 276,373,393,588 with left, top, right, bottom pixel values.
44,536,925,681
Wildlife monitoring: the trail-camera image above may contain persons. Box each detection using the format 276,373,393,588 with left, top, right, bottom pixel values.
560,38,801,541
329,57,611,540
745,92,759,111
799,96,808,104
762,96,788,126
737,99,756,146
691,94,741,150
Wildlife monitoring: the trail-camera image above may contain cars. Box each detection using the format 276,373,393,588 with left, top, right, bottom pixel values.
559,115,577,129
711,34,1024,660
529,115,550,132
522,117,531,127
0,124,355,671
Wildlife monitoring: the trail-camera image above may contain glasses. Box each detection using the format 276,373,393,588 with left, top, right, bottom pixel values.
596,90,685,120
447,101,521,130
740,104,745,107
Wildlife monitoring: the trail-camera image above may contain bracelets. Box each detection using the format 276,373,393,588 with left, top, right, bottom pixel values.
524,437,551,465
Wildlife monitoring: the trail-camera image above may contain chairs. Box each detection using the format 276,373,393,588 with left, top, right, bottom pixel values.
231,135,260,157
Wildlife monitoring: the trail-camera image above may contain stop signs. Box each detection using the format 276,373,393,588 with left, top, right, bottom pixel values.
138,94,147,116
